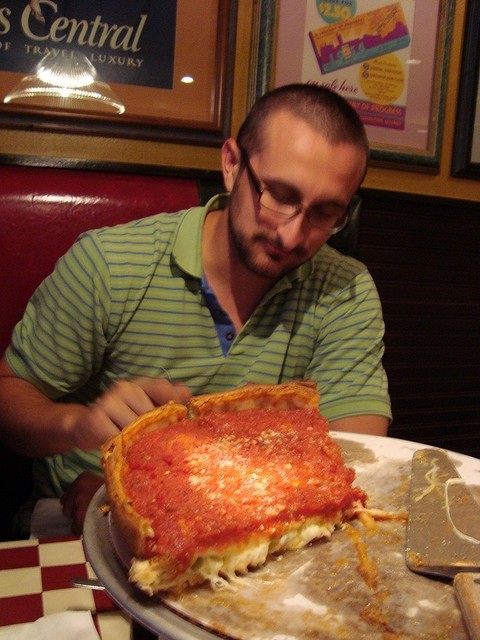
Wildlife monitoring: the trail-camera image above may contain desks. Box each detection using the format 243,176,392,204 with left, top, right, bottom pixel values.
2,534,163,638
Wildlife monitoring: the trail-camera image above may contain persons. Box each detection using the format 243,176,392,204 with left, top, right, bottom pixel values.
0,83,395,539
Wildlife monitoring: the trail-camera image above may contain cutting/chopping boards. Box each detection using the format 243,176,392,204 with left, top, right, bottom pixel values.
83,430,479,640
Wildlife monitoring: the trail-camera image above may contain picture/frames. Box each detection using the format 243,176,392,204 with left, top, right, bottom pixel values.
253,1,456,178
449,0,480,183
0,1,240,148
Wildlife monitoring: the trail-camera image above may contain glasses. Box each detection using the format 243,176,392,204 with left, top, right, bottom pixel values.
241,146,351,236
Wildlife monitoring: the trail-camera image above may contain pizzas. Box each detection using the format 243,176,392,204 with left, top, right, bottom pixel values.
101,377,366,595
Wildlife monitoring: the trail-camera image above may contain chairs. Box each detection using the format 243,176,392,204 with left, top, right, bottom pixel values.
1,165,202,361
